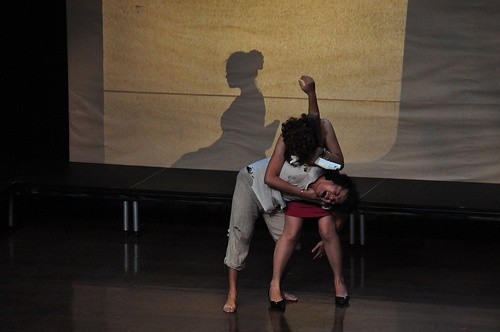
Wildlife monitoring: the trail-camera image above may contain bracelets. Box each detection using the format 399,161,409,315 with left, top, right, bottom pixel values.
300,189,306,198
319,150,331,160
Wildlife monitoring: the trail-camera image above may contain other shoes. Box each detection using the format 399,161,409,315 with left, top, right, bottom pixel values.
335,296,351,305
268,289,286,309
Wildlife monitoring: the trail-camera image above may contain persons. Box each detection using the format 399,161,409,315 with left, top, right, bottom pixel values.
223,75,360,313
264,113,350,310
